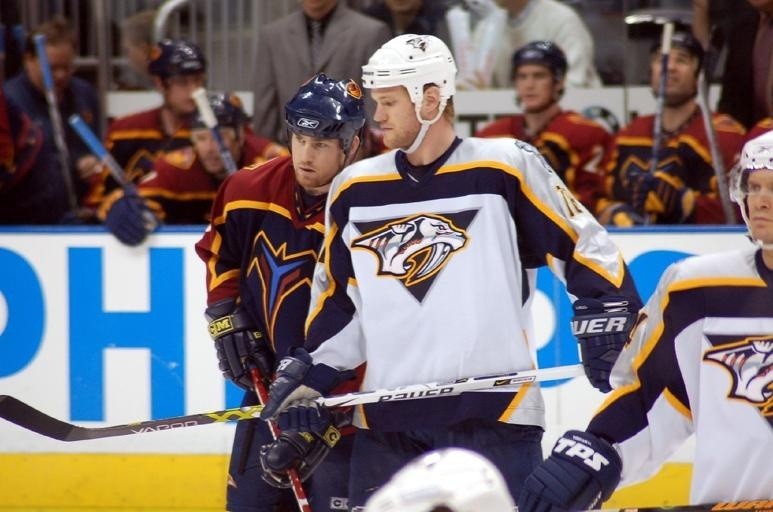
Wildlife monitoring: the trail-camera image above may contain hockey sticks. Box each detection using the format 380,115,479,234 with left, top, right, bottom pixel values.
0,362,585,441
624,13,675,215
696,26,739,224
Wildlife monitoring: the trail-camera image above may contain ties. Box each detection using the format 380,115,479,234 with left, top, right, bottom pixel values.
310,20,322,66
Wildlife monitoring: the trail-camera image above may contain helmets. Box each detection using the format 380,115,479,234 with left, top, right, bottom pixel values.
514,42,566,81
148,38,204,74
728,130,773,201
361,33,457,104
284,73,365,138
177,94,252,131
650,31,704,62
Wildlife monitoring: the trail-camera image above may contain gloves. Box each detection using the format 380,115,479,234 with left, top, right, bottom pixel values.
105,197,157,245
518,428,623,511
571,294,638,393
203,298,358,490
597,199,644,226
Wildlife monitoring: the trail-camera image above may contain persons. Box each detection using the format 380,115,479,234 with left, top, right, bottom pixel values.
193,74,370,511
0,1,773,248
255,33,644,510
518,128,773,511
